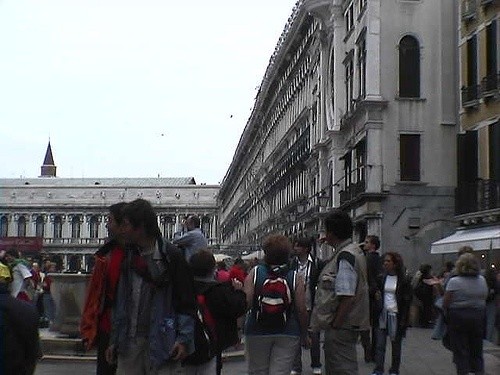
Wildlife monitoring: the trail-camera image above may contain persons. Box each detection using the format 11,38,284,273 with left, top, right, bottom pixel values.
0,247,53,375
79,199,500,375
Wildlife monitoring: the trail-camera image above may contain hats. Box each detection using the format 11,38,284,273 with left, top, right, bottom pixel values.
0,263,11,279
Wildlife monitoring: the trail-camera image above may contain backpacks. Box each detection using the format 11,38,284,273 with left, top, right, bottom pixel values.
253,267,295,325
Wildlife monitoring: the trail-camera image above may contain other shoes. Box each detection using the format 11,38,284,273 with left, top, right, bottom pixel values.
390,368,399,373
291,371,301,375
313,367,321,374
372,370,384,375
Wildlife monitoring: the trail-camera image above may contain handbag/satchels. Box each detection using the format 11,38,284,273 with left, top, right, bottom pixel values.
168,310,216,365
368,277,382,312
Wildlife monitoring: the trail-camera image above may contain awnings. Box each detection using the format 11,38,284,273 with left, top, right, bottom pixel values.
430,224,500,254
